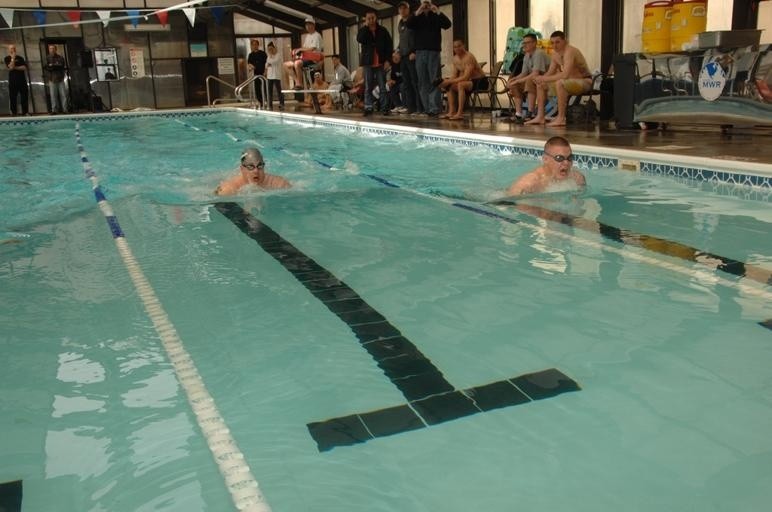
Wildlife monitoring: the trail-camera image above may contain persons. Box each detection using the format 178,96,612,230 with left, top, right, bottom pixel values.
247,39,268,108
501,34,551,124
262,41,285,109
44,45,71,115
4,44,32,116
104,68,116,80
504,136,587,224
216,147,293,198
523,31,594,128
437,39,489,120
284,0,452,120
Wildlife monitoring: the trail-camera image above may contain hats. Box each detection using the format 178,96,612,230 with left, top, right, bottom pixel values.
305,15,316,25
240,148,263,165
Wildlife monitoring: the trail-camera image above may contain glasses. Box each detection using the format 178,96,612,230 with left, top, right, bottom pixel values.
242,162,265,171
545,152,574,163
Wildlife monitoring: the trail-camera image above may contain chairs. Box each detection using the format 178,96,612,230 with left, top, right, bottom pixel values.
440,61,508,120
292,48,324,89
507,70,611,133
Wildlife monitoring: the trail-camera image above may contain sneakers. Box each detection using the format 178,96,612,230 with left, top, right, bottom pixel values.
364,104,443,119
11,111,71,117
500,111,536,125
291,86,304,91
259,103,285,111
